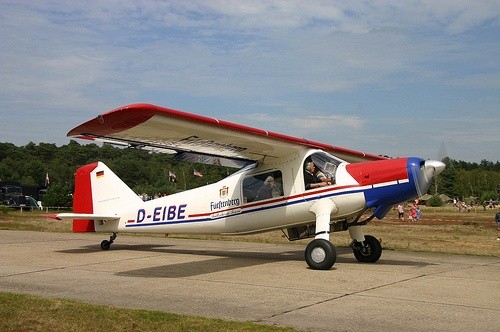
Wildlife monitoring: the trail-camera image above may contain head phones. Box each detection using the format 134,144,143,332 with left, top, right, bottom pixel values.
306,161,311,171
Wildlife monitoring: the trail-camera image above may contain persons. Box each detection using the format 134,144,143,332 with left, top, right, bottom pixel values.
408,199,421,223
304,158,336,190
495,208,500,241
453,197,478,213
243,175,283,204
483,201,487,211
398,203,405,220
489,201,492,210
137,193,167,202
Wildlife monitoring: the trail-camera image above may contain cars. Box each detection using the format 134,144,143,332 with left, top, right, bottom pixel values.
0,185,48,211
495,211,500,239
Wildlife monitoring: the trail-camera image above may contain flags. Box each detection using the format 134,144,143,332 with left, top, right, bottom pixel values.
169,171,177,183
194,168,203,177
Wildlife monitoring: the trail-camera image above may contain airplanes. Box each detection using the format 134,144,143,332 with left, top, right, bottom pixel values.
43,103,446,271
